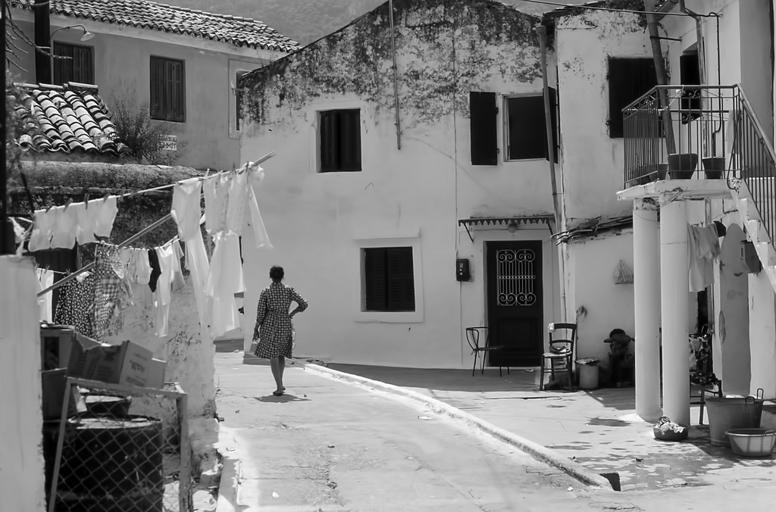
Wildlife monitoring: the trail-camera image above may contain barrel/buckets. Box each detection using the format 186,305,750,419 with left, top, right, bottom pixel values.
703,387,765,447
42,412,163,510
81,391,131,416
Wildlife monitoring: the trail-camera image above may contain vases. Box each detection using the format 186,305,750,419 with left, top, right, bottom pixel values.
631,153,725,183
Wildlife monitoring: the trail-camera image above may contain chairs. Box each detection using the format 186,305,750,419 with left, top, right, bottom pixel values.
465,327,511,376
541,323,577,391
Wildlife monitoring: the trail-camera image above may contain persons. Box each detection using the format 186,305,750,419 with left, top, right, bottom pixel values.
251,264,309,396
